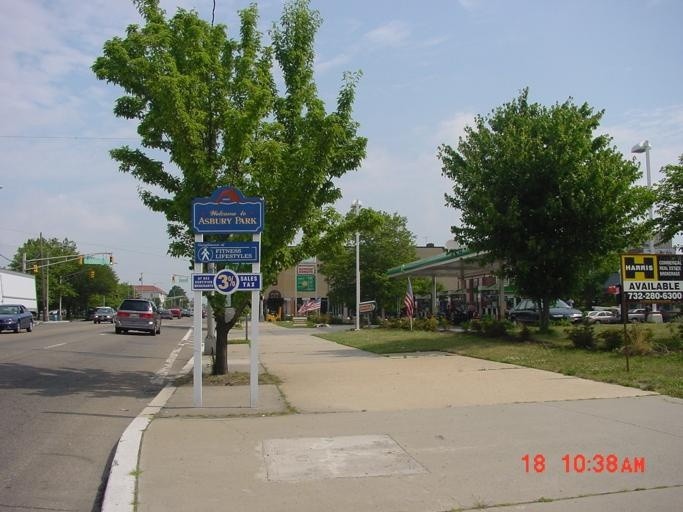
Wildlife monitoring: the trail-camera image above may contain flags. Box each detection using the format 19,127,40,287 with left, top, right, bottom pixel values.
306,297,321,311
298,302,308,313
404,280,414,319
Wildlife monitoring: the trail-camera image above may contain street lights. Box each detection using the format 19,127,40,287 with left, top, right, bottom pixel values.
0,185,3,189
134,271,145,301
350,198,364,333
630,138,662,313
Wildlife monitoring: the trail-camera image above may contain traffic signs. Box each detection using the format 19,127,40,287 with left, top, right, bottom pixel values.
84,256,106,264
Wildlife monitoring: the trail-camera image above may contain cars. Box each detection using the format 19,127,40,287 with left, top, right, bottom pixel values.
156,305,208,320
0,303,35,335
504,293,680,328
85,306,118,325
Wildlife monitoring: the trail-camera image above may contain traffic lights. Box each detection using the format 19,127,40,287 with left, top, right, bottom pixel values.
109,255,113,265
89,270,95,279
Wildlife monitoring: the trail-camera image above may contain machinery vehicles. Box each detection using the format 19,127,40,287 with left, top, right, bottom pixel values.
265,302,282,322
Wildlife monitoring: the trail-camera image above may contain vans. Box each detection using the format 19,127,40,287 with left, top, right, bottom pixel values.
114,299,163,336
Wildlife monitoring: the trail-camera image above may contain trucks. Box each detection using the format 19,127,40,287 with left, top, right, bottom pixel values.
0,268,38,319
